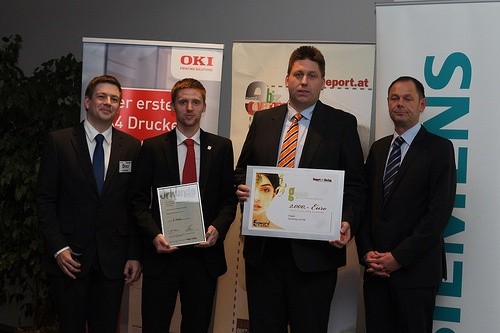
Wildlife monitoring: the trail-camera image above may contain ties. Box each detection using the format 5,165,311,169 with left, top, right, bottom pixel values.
278,113,303,168
93,134,104,198
383,136,405,208
182,139,197,184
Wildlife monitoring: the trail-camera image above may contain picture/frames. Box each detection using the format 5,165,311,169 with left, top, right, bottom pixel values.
157,181,206,247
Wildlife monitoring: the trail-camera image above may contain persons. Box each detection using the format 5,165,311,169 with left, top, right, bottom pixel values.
34,73,148,332
231,45,367,331
129,76,241,332
251,172,288,229
351,75,458,333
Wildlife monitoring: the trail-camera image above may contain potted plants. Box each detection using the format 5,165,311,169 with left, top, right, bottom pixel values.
0,32,83,333
